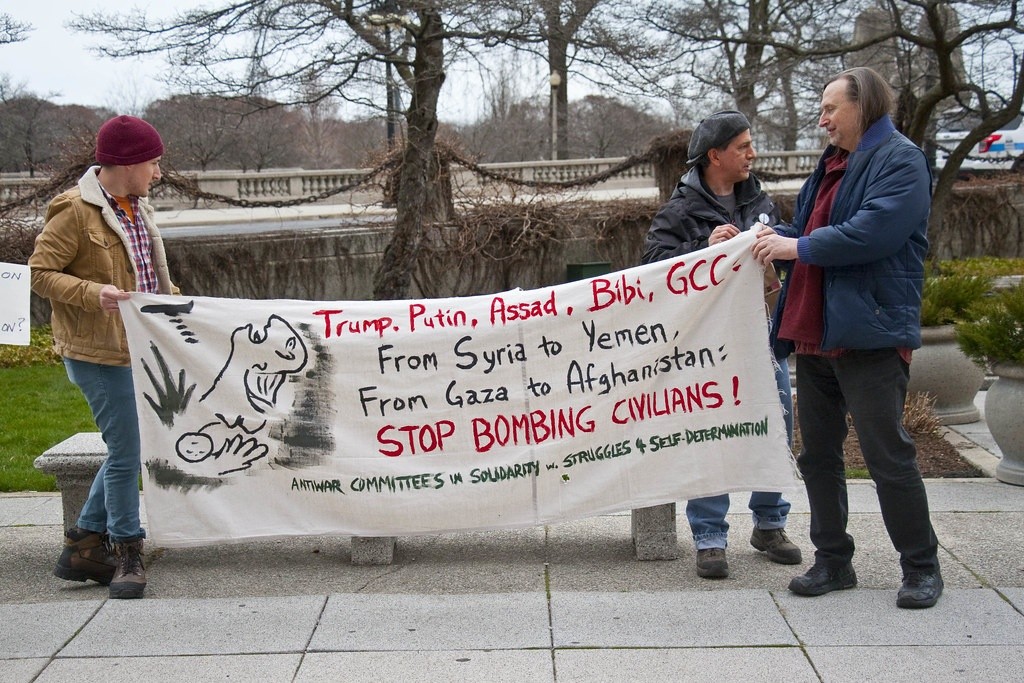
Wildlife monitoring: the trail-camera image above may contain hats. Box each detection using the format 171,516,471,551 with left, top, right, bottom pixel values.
685,110,751,164
95,115,164,166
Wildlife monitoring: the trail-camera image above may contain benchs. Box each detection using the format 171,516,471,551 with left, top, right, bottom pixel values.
34,433,679,560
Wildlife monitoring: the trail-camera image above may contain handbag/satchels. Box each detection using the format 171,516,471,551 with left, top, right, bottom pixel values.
764,262,783,319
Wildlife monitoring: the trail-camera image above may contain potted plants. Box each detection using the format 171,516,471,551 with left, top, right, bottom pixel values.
951,277,1023,484
909,267,991,426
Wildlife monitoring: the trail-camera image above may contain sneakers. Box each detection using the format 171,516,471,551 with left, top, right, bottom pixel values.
788,560,857,596
696,548,730,578
896,570,944,609
52,531,119,585
750,523,802,565
109,538,147,598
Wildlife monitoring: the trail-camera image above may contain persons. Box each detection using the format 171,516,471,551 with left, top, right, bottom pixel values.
641,110,801,580
750,67,943,611
27,115,182,598
